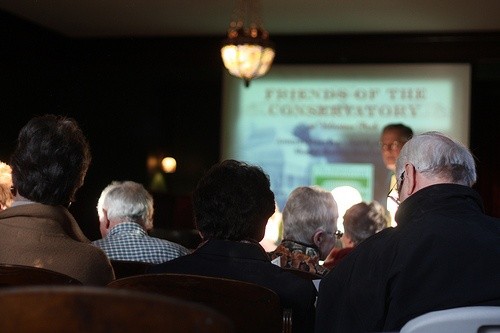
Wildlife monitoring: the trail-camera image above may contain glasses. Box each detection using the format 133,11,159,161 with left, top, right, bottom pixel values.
386,163,414,203
330,230,343,239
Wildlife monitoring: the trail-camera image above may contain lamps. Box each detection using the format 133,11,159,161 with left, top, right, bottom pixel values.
219,1,278,85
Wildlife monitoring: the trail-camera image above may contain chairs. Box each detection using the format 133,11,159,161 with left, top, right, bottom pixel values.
0,262,296,333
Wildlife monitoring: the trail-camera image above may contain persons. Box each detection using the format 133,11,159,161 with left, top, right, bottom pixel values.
88,181,191,267
0,114,117,288
268,186,391,293
0,161,14,212
379,122,414,191
315,131,500,333
148,157,325,304
